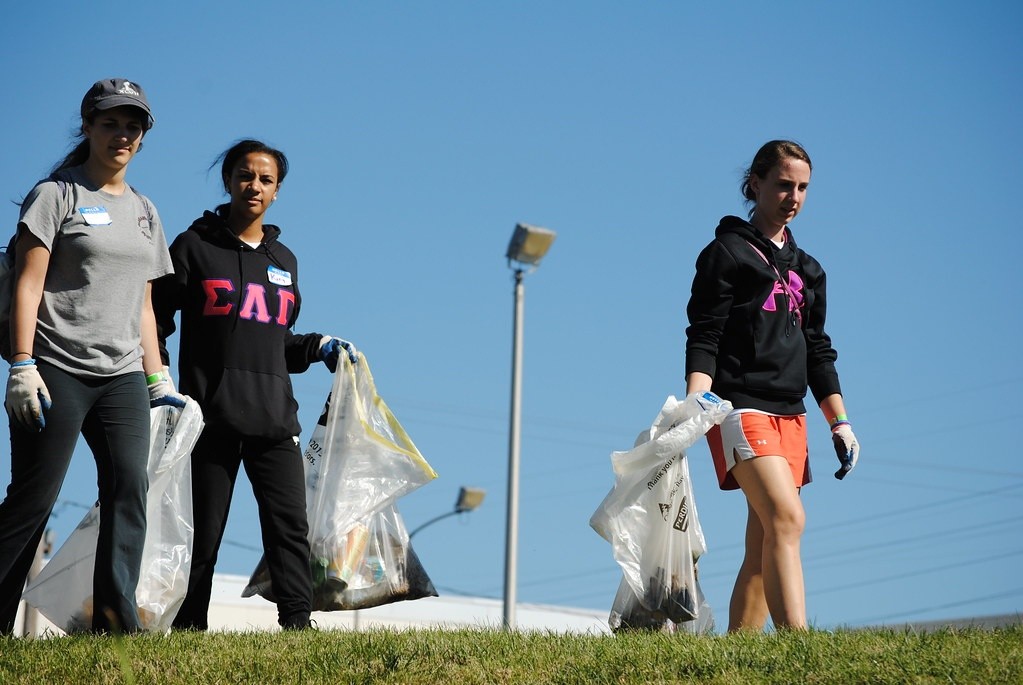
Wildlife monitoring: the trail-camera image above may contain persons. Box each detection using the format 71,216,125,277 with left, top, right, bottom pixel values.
684,139,860,635
151,139,357,632
0,78,175,636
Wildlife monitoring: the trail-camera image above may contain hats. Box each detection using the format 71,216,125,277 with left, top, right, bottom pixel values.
80,78,155,122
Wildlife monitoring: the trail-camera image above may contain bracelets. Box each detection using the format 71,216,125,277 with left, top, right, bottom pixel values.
11,352,33,358
827,414,848,427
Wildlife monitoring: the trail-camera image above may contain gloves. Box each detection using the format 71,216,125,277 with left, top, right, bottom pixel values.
4,365,52,433
147,378,187,413
832,426,860,480
320,334,359,374
161,364,177,392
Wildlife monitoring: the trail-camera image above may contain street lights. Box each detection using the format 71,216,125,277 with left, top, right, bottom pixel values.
502,224,558,634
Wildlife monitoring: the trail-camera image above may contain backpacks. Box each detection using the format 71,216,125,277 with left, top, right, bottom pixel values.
0,175,150,364
